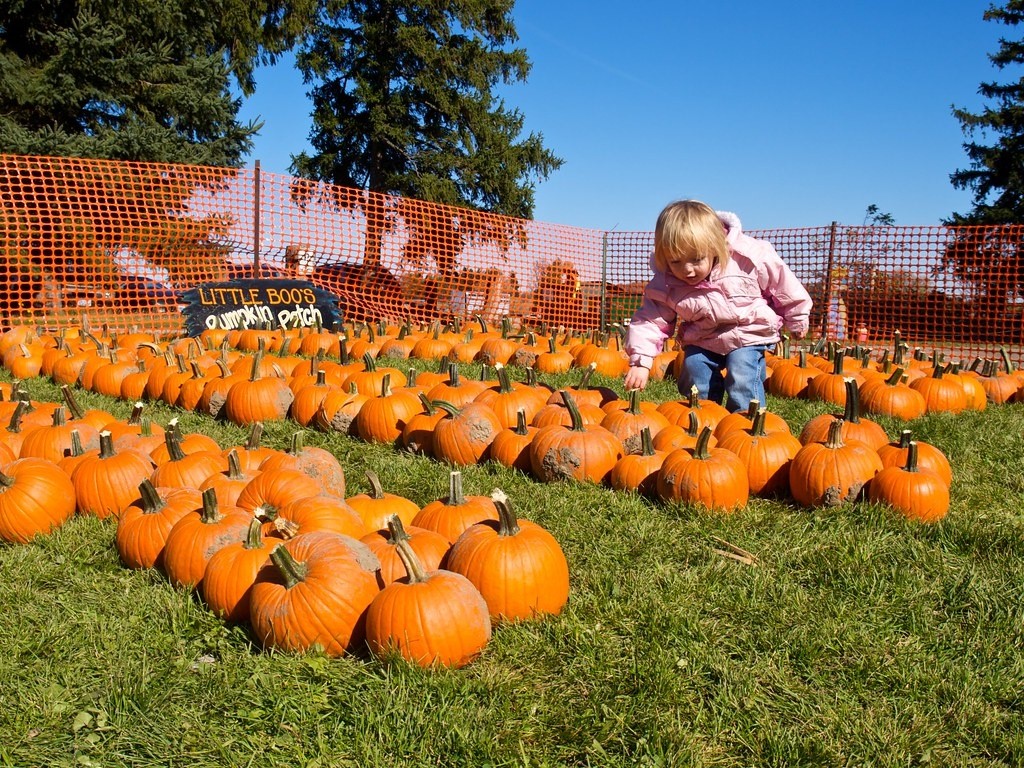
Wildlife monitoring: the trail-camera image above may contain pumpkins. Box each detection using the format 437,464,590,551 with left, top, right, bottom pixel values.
0,322,952,523
202,310,1024,422
0,375,571,668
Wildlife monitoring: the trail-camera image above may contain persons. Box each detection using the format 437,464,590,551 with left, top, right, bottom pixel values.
857,323,867,342
622,200,813,413
